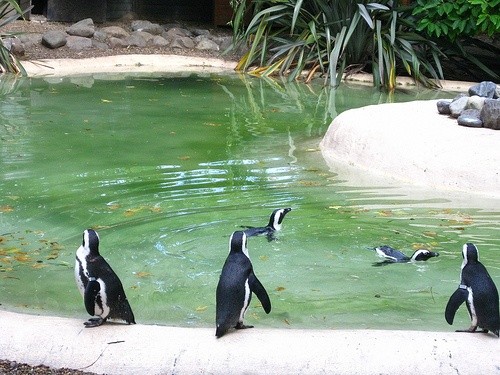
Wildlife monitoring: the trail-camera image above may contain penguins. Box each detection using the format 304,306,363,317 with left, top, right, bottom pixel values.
238,208,292,242
73,228,135,328
214,230,271,339
444,242,500,338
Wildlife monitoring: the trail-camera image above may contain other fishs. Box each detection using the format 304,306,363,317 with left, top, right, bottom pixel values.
88,199,160,231
258,255,269,260
98,73,333,187
1,119,74,279
131,285,139,291
275,284,335,326
136,272,151,279
328,198,473,250
196,305,208,314
